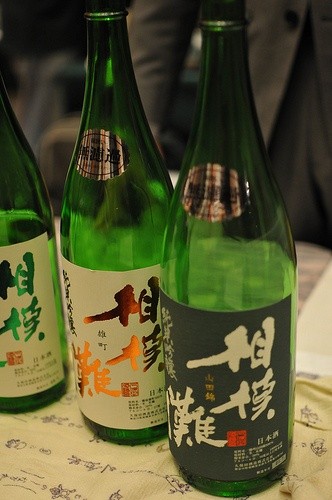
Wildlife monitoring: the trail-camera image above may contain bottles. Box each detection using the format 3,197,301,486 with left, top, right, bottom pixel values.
1,77,70,416
159,19,299,500
57,11,186,447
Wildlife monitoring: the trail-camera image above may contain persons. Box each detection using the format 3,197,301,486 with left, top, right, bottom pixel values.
129,0,332,248
